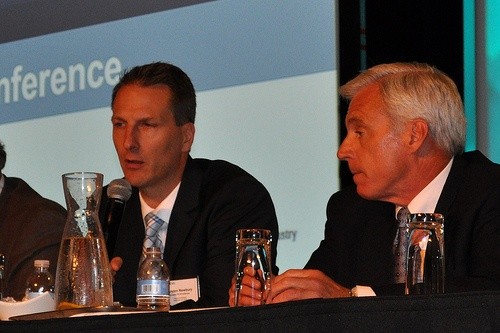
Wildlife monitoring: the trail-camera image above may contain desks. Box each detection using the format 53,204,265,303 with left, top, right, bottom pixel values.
0,290,500,333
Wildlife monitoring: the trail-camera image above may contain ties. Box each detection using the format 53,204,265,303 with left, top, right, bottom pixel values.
142,213,165,263
391,207,411,284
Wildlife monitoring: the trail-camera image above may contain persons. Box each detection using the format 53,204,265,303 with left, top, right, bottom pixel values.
67,62,279,310
0,144,74,302
228,63,500,306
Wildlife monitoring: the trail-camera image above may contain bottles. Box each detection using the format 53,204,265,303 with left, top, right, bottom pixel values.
135,247,171,312
52,171,114,310
26,260,54,300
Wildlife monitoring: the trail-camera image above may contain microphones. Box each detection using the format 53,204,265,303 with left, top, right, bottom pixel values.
105,179,133,256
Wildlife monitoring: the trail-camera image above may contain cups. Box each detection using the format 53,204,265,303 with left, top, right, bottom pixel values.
405,213,446,294
235,229,273,307
0,254,6,301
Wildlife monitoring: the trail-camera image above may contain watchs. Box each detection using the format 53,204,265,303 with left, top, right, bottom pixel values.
348,287,358,298
